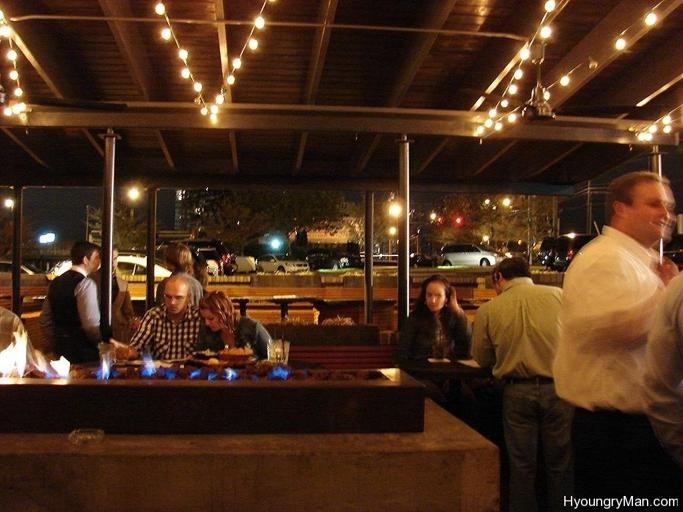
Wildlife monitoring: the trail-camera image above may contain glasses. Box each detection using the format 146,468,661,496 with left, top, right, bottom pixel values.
164,293,185,300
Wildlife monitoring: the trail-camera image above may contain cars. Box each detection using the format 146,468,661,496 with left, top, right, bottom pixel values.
410,240,529,268
0,243,364,276
663,235,683,271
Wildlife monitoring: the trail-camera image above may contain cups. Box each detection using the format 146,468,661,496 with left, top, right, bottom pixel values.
267,340,290,366
432,344,447,360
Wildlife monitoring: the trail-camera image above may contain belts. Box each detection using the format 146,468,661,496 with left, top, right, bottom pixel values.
504,376,553,384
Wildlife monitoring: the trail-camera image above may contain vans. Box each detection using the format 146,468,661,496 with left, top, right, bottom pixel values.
537,233,598,272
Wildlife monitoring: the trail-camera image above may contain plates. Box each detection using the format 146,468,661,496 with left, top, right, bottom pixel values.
190,352,254,360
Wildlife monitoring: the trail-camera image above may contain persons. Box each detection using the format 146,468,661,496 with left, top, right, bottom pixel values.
196,291,274,361
641,269,683,512
37,240,103,365
470,257,573,512
191,245,238,290
90,245,135,345
109,275,201,361
154,245,204,319
398,274,479,426
552,171,680,512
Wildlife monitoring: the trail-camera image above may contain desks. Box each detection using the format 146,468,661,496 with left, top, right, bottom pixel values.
396,359,496,415
